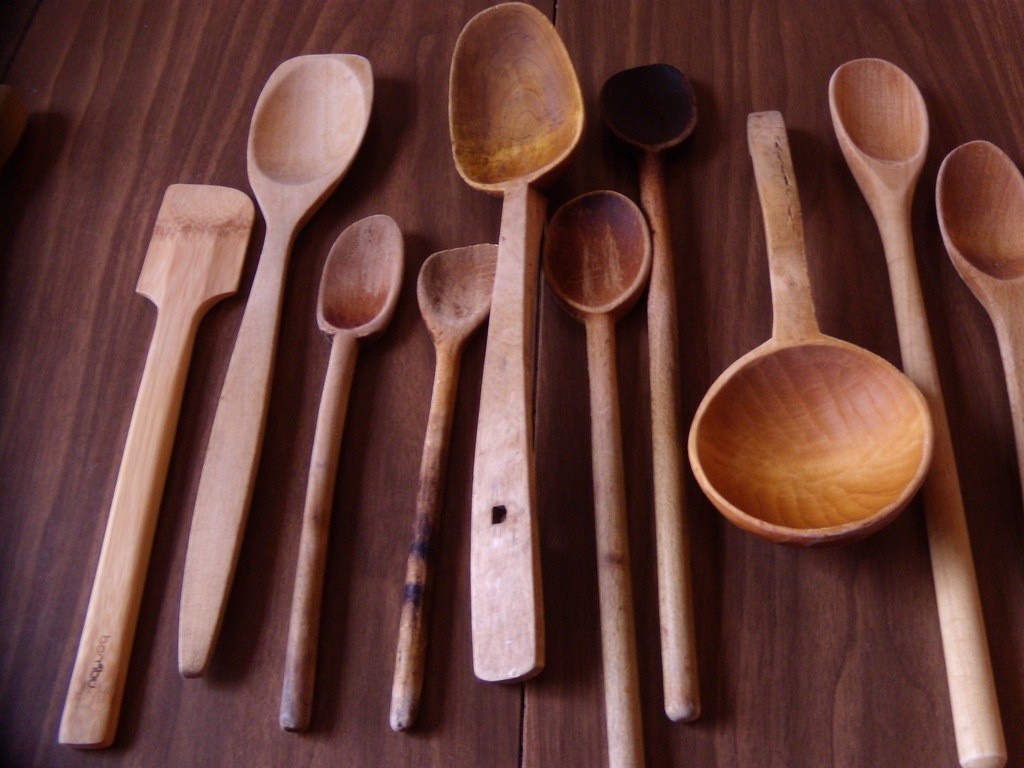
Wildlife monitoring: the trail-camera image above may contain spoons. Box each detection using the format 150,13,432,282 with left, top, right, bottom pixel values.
604,64,699,721
830,57,1010,768
542,189,650,768
279,214,403,730
389,244,499,733
937,141,1024,495
